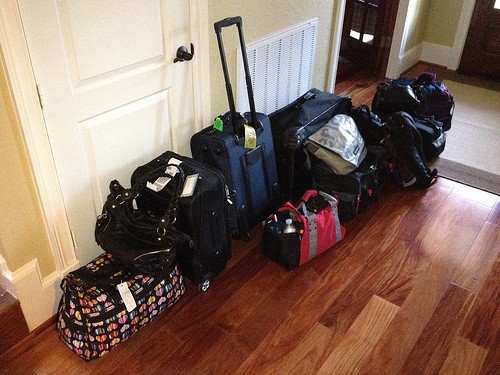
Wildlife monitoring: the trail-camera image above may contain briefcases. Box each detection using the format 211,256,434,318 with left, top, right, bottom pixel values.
268,88,354,201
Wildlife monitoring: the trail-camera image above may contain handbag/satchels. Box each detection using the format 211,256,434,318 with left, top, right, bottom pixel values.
303,114,368,175
94,164,185,277
55,252,187,361
259,190,346,270
352,72,455,161
309,146,389,221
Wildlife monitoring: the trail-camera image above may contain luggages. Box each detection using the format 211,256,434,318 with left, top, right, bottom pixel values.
131,151,233,293
190,16,282,242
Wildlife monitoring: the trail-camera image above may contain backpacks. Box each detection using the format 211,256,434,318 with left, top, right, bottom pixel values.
379,111,438,190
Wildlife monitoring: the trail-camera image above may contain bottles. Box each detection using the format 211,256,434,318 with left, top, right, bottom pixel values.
282,219,296,233
